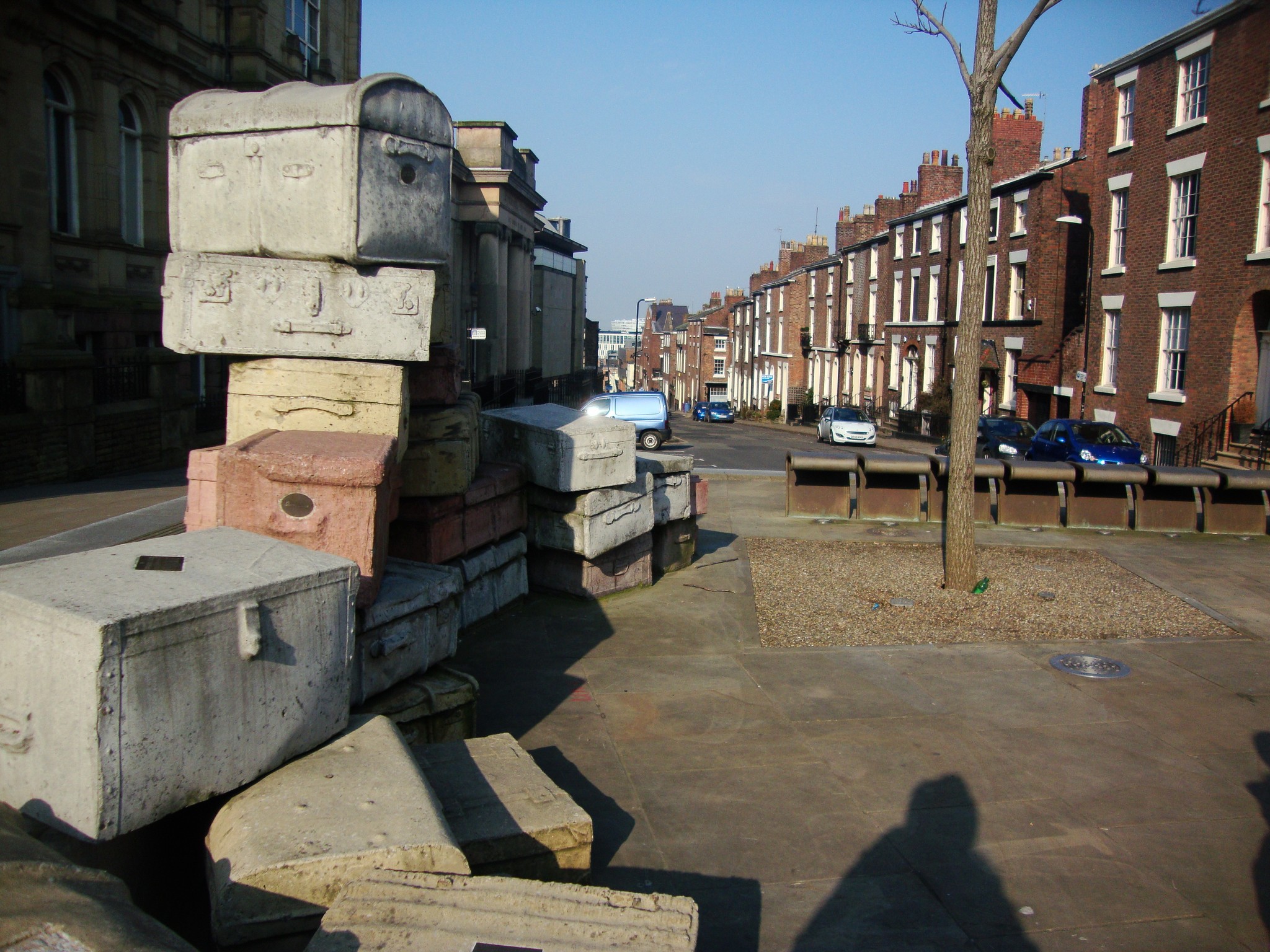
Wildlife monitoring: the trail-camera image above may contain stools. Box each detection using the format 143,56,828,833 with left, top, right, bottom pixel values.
785,451,1270,535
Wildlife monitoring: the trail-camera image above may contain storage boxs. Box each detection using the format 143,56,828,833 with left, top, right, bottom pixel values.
0,76,700,952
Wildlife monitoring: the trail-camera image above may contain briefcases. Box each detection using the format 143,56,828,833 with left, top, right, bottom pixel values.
0,73,708,952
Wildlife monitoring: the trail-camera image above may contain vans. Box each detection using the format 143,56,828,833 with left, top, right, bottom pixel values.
577,391,673,451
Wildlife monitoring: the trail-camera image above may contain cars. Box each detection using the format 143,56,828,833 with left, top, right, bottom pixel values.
692,402,710,421
704,402,734,424
816,407,876,447
946,414,1039,460
1024,418,1150,466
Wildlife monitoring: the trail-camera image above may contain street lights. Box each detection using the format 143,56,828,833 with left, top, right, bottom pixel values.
1056,216,1095,419
633,298,657,391
655,371,661,391
